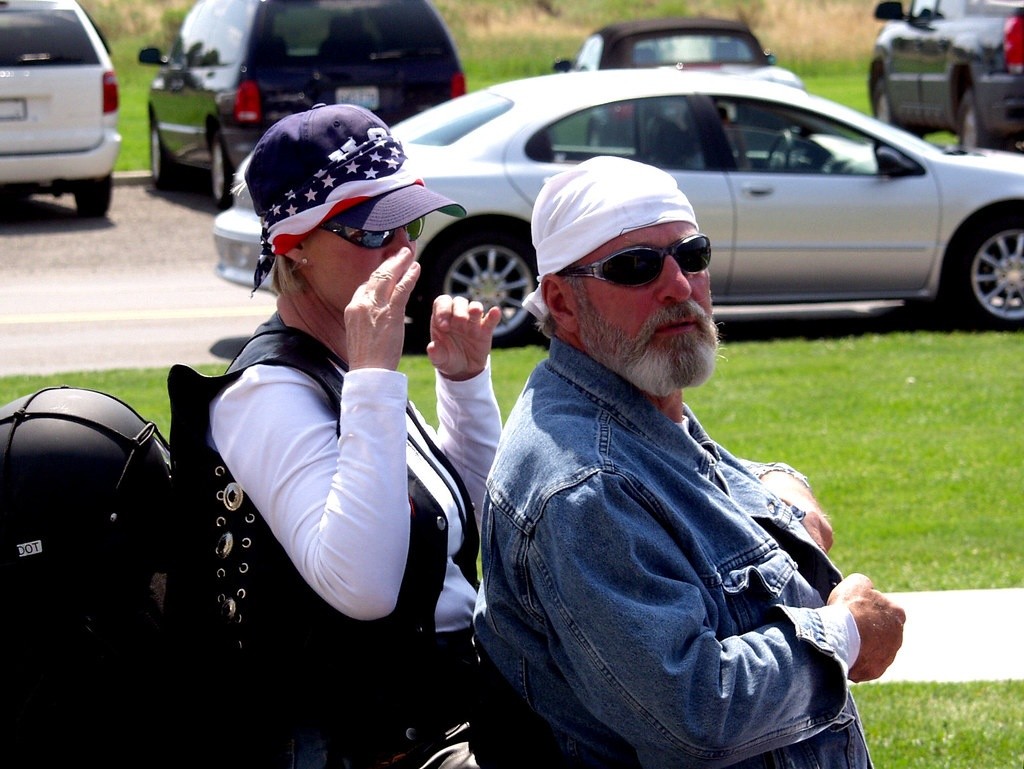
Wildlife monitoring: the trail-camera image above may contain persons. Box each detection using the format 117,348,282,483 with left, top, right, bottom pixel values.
470,154,906,769
167,104,502,769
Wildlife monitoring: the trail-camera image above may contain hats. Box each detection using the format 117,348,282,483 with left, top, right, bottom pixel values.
241,103,466,256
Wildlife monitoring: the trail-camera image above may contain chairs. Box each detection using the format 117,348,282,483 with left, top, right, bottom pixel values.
626,41,746,63
526,115,724,171
264,12,375,66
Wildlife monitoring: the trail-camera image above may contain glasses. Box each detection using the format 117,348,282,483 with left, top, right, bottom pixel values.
316,213,423,251
557,231,710,291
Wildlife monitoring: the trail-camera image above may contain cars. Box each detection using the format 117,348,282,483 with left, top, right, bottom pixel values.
138,0,467,214
866,1,1023,155
0,0,123,221
210,67,1024,349
550,17,775,148
